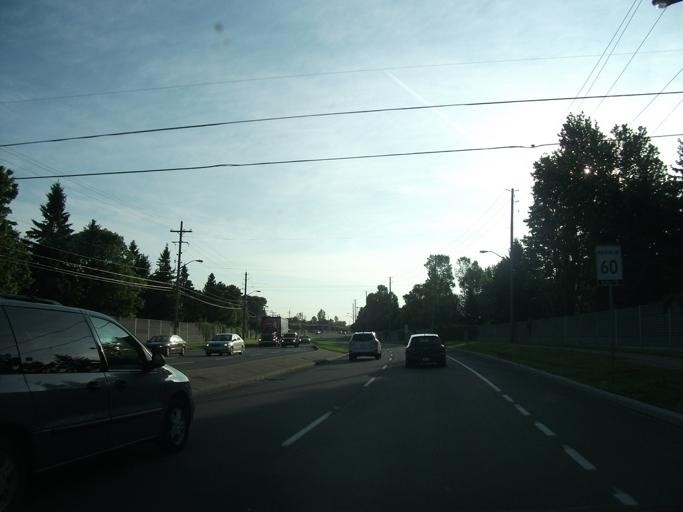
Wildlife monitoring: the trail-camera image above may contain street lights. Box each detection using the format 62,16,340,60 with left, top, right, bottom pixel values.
480,250,516,344
242,290,261,342
174,260,203,335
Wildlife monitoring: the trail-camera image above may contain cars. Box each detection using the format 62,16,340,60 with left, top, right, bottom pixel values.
261,330,312,348
145,334,187,358
405,333,447,369
203,333,246,356
0,293,196,512
348,331,382,361
